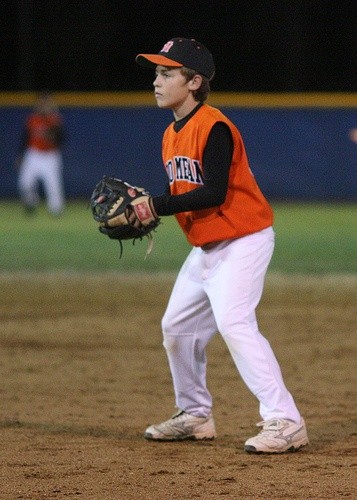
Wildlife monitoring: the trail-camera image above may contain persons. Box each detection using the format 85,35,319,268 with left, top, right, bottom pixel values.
14,94,70,217
103,37,310,454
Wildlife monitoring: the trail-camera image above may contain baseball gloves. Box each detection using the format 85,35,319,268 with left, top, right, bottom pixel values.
89,174,162,241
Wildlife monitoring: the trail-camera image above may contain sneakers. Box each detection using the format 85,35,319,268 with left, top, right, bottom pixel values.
143,408,217,442
243,417,309,455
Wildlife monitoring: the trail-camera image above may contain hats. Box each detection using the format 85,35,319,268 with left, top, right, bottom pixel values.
135,37,217,82
